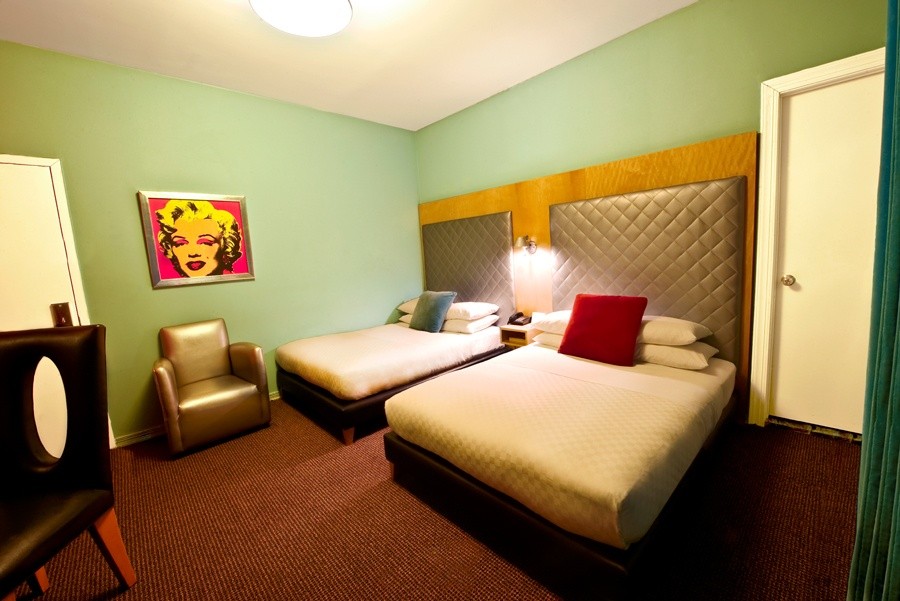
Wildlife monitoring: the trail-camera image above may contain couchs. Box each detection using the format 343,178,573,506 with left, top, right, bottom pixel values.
152,317,272,456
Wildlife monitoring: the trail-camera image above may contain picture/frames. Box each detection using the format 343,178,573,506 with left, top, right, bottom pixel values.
138,190,255,289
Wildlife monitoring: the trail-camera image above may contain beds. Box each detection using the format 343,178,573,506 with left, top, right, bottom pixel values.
272,324,509,446
383,344,737,601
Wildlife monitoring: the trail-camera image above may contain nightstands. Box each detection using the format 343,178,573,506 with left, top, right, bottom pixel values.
500,325,543,350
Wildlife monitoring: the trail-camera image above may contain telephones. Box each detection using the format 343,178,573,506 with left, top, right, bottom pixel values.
509,312,531,326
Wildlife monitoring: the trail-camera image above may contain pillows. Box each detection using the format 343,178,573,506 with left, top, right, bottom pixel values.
531,310,572,334
636,315,713,347
633,341,720,370
397,298,420,314
399,312,412,324
440,315,499,333
445,302,501,321
557,293,647,367
531,332,563,346
409,291,457,333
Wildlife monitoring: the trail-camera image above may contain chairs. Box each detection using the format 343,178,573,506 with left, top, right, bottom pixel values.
0,323,139,601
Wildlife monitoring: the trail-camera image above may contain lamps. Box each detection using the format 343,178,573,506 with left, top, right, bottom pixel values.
513,233,538,255
